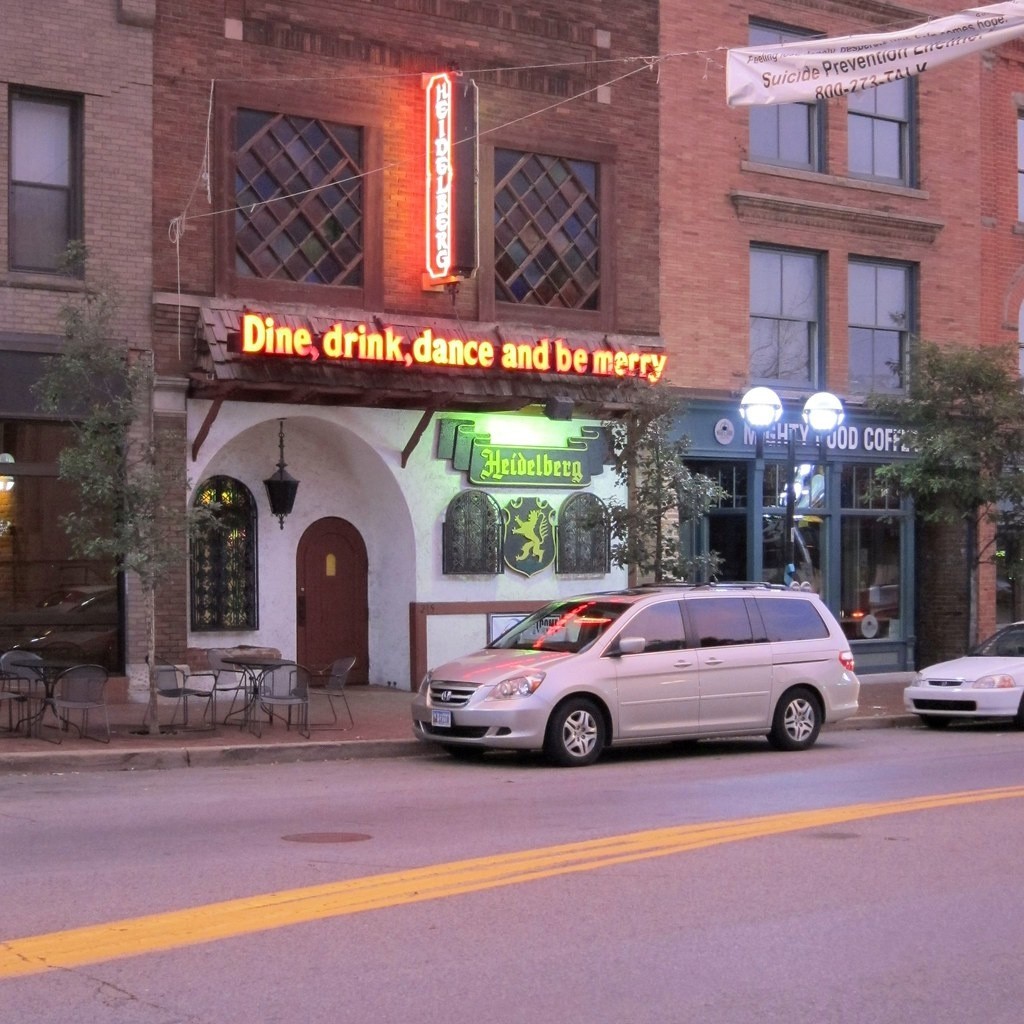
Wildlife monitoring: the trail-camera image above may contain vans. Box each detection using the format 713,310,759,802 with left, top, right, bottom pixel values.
410,580,861,767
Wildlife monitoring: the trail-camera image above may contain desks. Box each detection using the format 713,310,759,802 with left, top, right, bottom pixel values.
10,661,81,739
223,657,294,738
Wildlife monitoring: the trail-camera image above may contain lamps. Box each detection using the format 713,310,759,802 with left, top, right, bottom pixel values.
262,417,300,530
0,476,15,491
544,396,576,422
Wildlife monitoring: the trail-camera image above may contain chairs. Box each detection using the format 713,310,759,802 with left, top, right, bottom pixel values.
0,647,356,743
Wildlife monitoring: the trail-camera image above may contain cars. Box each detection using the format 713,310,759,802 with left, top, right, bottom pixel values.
903,621,1024,733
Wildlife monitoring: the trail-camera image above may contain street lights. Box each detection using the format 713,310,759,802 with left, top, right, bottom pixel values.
739,385,846,585
